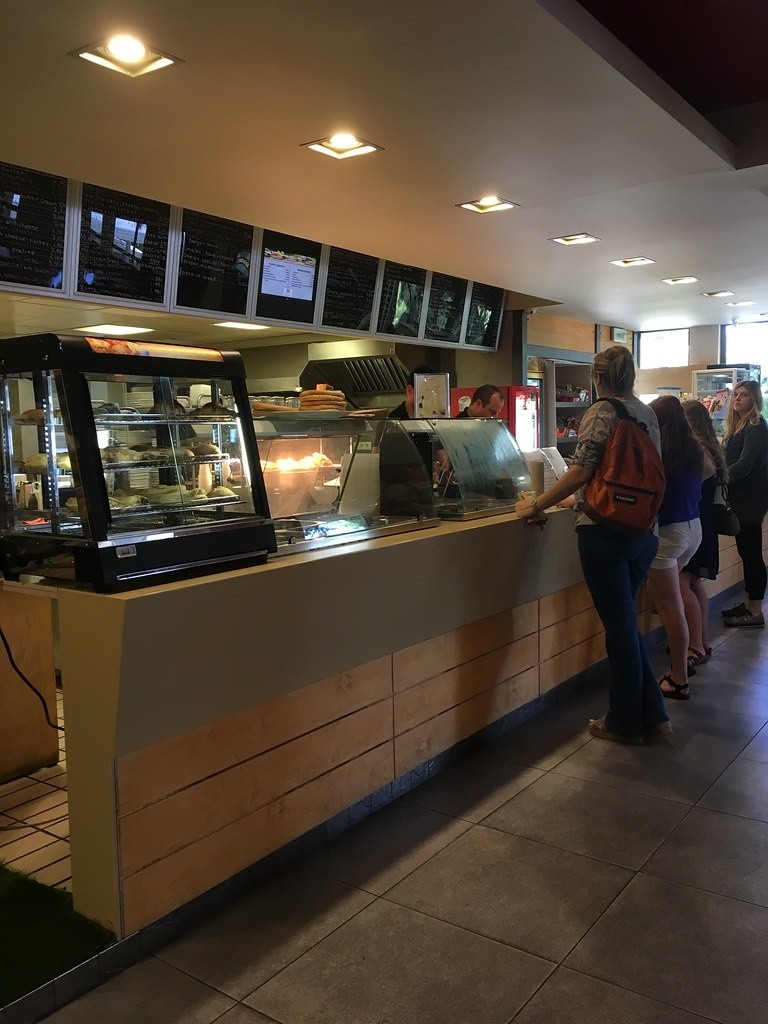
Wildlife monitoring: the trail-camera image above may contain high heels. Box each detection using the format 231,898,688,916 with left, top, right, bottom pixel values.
644,720,673,736
589,714,643,744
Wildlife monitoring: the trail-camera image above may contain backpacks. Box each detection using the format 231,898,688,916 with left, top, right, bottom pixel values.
580,397,666,538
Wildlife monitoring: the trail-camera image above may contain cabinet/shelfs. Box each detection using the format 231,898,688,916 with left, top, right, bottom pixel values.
548,360,595,451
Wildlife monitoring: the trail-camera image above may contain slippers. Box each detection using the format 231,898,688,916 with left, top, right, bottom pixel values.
687,647,708,665
705,647,712,655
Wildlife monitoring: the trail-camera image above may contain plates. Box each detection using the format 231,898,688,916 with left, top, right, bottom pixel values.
265,412,349,419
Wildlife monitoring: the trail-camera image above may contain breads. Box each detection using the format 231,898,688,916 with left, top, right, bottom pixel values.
18,402,236,509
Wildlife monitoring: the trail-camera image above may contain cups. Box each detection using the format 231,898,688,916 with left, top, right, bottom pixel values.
222,395,299,413
198,464,212,494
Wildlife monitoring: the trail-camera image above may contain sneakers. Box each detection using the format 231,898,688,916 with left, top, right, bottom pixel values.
723,609,765,628
721,602,748,617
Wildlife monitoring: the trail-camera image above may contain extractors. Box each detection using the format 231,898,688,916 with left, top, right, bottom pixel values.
172,338,456,398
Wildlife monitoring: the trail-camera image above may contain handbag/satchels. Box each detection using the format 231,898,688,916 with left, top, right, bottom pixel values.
713,483,743,536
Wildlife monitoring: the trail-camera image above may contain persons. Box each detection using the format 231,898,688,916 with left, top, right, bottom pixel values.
720,381,768,628
679,399,729,666
515,345,674,743
647,396,715,700
455,384,505,418
372,365,437,453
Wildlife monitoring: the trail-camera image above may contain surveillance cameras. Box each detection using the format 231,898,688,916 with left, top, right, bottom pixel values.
529,308,536,315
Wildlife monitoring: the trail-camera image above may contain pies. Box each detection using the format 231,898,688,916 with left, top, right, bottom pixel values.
252,389,347,412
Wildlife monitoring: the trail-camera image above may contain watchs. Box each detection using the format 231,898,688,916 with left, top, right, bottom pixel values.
531,498,539,512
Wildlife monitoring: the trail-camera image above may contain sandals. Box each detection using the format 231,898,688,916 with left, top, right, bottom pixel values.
662,664,696,677
658,675,689,700
